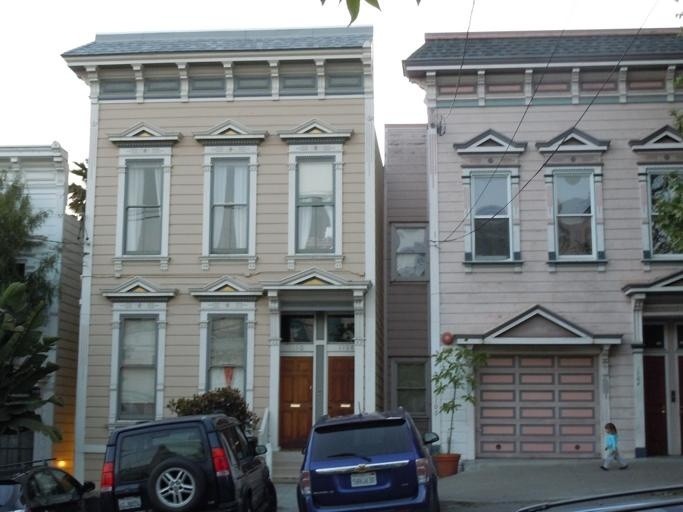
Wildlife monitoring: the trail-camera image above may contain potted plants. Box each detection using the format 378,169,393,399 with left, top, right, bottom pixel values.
428,339,488,477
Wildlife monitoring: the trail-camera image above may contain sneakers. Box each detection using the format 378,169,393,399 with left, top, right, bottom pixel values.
618,463,630,471
600,465,610,471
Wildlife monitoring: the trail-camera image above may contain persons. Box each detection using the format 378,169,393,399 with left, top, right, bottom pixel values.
599,422,628,471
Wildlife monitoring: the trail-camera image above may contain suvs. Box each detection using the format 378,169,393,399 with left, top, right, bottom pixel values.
292,405,442,512
95,409,278,510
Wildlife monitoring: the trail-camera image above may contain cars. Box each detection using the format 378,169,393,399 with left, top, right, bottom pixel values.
0,456,102,512
